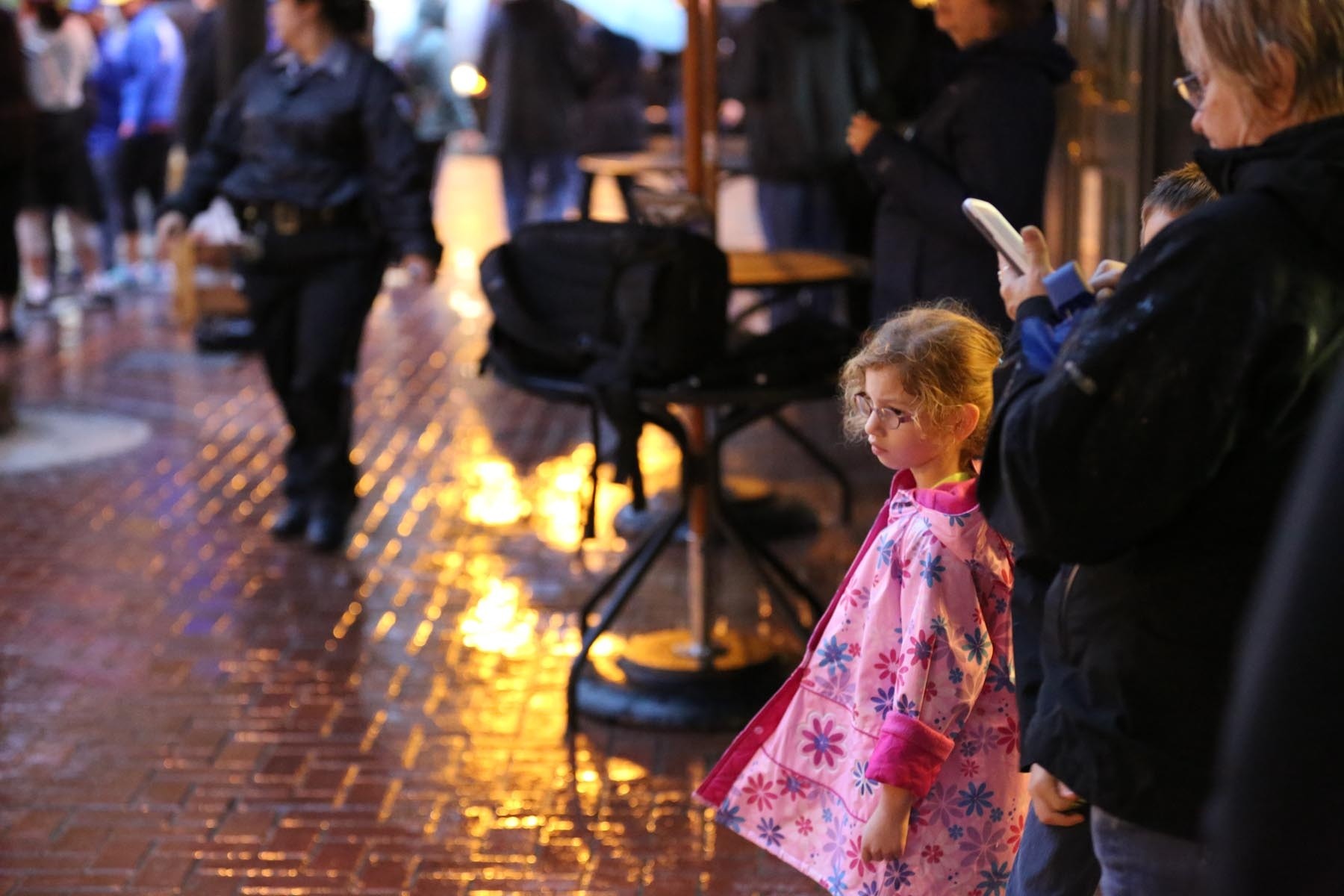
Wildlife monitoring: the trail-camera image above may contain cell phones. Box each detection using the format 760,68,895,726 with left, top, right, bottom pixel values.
963,197,1037,275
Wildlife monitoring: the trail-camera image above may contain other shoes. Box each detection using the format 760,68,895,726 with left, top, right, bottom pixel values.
270,503,350,550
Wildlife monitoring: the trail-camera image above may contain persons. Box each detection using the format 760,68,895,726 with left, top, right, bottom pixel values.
152,1,446,549
1,1,1344,896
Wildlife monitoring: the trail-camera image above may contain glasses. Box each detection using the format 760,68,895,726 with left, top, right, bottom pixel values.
853,390,912,429
1174,73,1207,110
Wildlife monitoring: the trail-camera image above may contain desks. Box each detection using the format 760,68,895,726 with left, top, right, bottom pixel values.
613,248,855,694
577,149,757,224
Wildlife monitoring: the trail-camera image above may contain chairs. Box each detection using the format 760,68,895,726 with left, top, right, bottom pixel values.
481,224,855,745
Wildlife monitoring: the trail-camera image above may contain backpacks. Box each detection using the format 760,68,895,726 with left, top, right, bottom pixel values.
480,218,731,386
